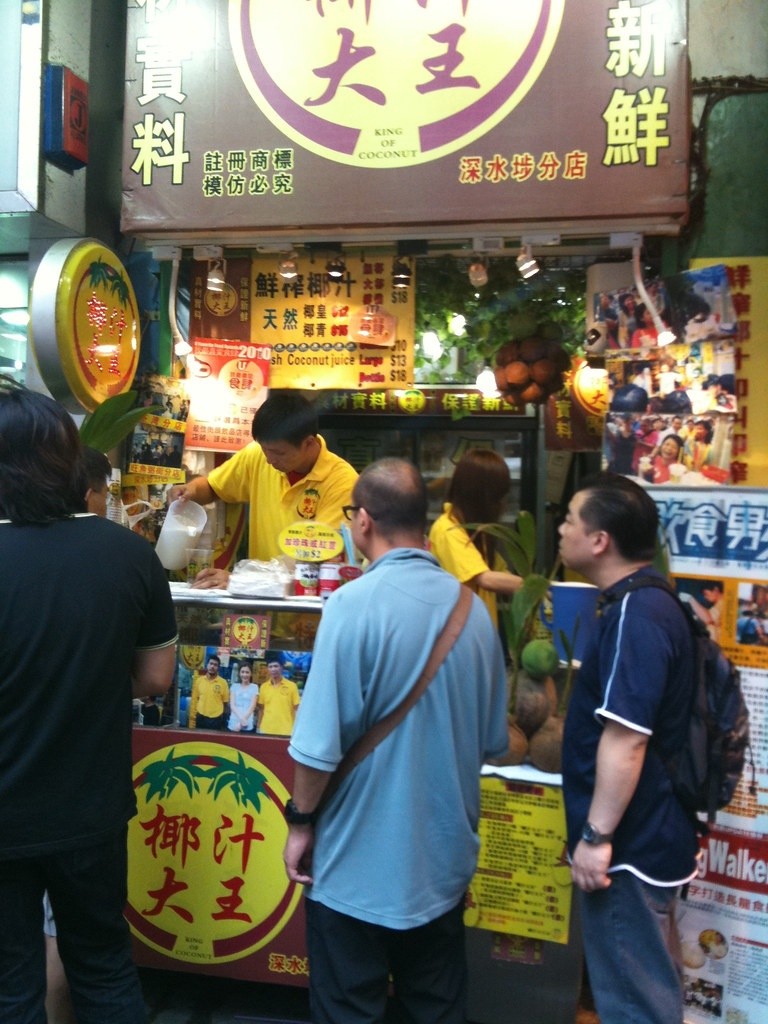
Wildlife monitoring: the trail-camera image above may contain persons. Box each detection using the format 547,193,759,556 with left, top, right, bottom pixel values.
557,472,702,1024
188,656,300,735
167,393,359,589
141,696,160,726
429,448,552,598
595,284,767,648
0,391,178,1024
283,459,511,1024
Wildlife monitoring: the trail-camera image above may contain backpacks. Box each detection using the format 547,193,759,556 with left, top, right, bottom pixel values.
598,578,750,838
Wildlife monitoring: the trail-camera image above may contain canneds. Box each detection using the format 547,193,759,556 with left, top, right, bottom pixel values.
293,561,341,599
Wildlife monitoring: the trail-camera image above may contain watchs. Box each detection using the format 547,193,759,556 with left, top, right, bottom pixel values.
285,799,315,825
581,822,613,844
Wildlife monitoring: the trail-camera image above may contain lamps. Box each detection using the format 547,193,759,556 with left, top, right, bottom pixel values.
515,234,562,279
320,242,347,277
468,237,505,288
192,245,228,293
390,240,430,288
609,232,678,348
152,246,194,356
277,244,311,281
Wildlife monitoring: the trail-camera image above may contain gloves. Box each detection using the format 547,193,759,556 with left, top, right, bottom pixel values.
679,591,693,604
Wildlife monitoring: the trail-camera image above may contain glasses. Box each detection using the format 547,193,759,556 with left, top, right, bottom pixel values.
342,506,378,521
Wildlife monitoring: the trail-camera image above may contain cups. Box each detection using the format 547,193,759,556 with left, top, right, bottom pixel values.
106,467,129,530
184,548,215,589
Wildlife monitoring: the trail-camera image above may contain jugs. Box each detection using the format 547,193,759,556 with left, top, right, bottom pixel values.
539,580,604,671
154,495,207,571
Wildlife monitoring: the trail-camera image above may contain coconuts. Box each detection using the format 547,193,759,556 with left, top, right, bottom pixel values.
489,314,571,407
498,667,567,773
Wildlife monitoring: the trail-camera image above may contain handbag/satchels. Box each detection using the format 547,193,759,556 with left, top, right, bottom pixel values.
741,617,758,643
